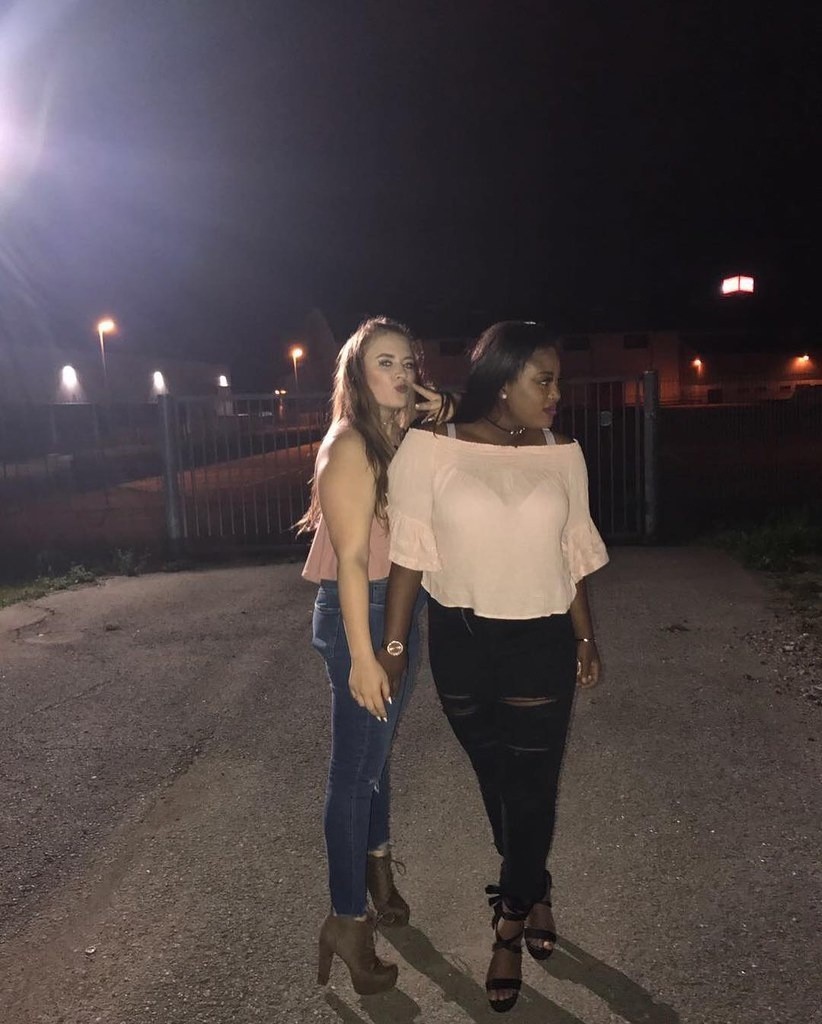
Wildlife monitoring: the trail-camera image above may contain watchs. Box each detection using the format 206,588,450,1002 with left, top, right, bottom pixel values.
381,639,408,657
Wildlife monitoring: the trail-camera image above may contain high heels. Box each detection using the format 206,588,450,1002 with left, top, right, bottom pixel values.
365,852,410,926
316,903,398,995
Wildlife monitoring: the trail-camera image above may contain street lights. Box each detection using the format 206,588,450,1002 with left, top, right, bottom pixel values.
718,274,754,411
292,347,304,389
96,320,114,399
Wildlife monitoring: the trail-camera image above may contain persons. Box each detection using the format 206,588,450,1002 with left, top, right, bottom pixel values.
290,316,471,996
373,321,609,1012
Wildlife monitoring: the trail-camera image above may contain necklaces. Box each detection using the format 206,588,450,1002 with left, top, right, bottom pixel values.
380,418,398,426
481,414,528,436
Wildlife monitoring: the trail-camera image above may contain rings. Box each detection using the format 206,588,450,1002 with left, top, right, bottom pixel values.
428,417,435,422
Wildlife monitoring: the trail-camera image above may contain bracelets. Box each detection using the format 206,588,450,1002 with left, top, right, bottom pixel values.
576,637,596,643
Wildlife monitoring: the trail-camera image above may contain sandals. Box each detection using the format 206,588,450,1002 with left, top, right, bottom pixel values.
522,897,556,960
485,908,523,1013
